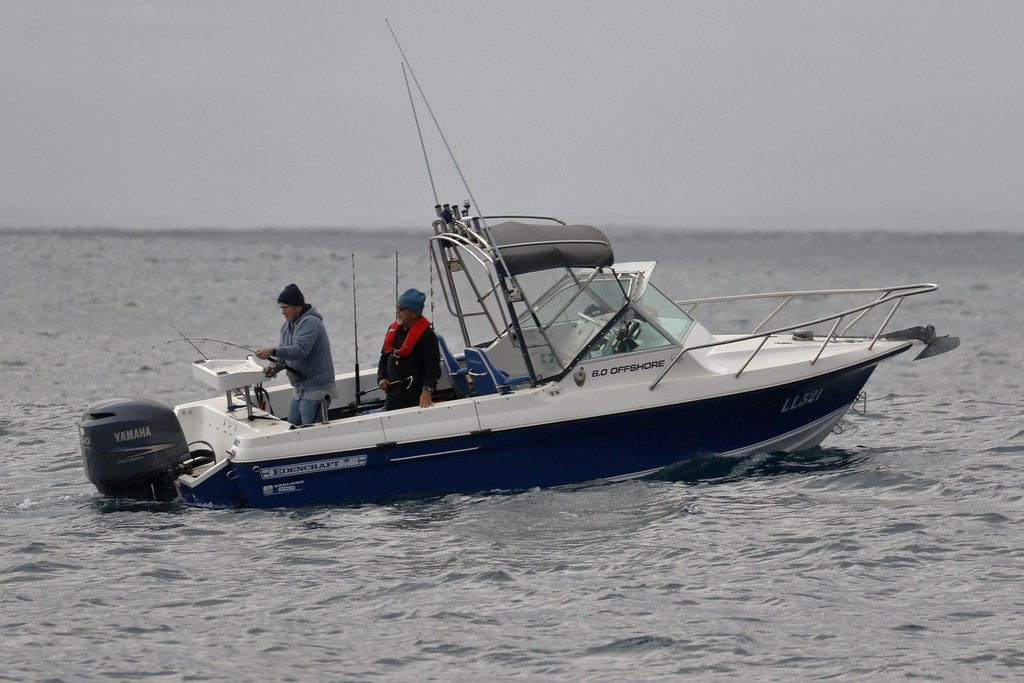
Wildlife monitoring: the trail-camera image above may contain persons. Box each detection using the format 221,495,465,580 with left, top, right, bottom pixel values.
254,283,338,426
377,288,442,411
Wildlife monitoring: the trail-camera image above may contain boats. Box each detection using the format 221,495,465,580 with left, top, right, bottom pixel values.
72,198,967,509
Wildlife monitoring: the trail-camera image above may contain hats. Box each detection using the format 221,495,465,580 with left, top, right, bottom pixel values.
396,288,426,314
277,284,304,306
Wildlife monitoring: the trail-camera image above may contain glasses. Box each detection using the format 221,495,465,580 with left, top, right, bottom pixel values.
396,305,408,312
278,305,296,310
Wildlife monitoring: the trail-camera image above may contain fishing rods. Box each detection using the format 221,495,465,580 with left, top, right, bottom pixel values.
126,336,305,384
